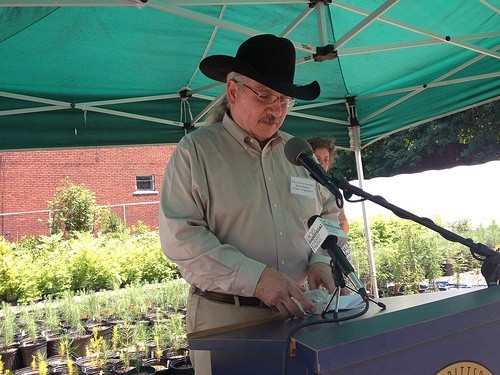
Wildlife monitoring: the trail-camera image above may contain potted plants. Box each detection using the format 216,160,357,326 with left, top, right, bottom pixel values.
0,274,196,375
346,219,500,300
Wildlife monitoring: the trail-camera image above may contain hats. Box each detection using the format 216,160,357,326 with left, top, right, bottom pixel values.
199,34,321,100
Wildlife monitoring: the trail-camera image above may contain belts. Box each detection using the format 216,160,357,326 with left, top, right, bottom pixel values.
191,284,266,307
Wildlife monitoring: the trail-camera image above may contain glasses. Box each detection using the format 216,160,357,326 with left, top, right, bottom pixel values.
235,80,297,108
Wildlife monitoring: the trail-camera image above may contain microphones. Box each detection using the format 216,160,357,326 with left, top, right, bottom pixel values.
284,136,343,200
303,215,368,302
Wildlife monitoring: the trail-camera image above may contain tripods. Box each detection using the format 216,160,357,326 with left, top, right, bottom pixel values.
320,261,387,319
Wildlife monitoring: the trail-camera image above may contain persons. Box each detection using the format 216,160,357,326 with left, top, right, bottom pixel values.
307,135,352,295
157,32,347,375
